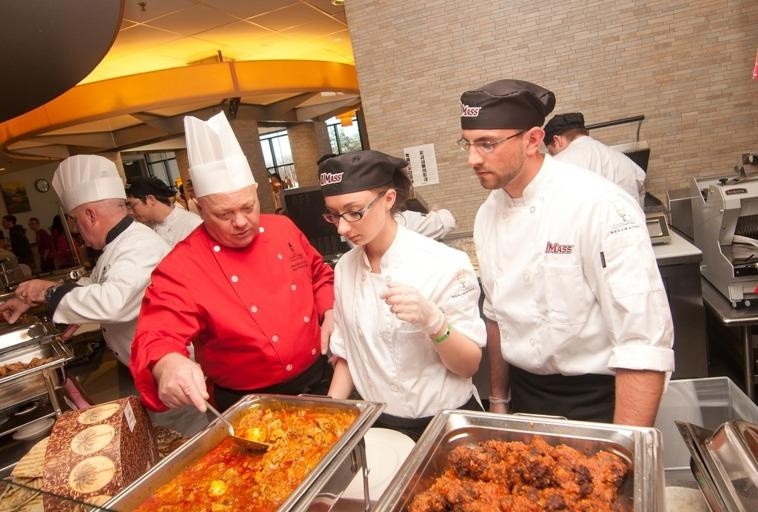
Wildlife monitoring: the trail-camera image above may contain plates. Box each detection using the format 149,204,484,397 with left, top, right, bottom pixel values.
13,416,56,441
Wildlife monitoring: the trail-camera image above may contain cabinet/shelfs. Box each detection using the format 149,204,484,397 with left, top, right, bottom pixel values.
696,271,757,401
0,367,67,493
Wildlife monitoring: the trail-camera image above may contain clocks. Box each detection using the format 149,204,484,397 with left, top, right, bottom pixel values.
33,175,54,195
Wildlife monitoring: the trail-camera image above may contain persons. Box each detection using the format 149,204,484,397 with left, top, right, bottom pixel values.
317,149,487,443
124,174,204,249
344,207,457,248
268,173,283,210
1,211,103,275
542,111,646,210
128,183,335,417
460,79,675,428
0,197,208,438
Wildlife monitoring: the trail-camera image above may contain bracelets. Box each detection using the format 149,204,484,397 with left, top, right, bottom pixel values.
429,323,453,344
487,396,511,405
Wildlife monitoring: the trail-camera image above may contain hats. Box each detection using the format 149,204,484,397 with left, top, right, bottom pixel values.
184,111,255,198
125,175,177,197
51,154,127,214
318,150,408,195
543,114,584,145
460,79,556,131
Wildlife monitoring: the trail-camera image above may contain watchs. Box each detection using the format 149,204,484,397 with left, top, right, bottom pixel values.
43,283,62,302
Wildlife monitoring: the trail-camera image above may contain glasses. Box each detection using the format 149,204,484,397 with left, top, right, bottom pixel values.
456,130,526,152
322,193,385,224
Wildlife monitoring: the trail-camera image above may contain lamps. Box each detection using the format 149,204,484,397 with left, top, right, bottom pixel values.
336,107,361,129
